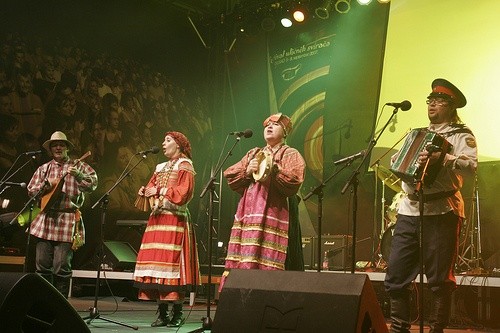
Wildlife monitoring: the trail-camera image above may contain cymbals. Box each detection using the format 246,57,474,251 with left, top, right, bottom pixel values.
378,164,403,193
254,152,271,182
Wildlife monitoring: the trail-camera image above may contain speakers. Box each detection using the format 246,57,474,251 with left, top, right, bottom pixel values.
102,239,139,272
0,271,91,333
212,267,388,333
301,235,372,270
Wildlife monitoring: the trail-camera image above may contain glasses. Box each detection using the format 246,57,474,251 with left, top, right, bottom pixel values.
426,100,453,106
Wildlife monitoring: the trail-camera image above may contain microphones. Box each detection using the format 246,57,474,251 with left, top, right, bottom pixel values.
22,150,46,156
3,182,26,189
334,151,366,165
426,144,440,151
385,100,411,111
229,129,252,138
344,119,352,139
136,148,160,155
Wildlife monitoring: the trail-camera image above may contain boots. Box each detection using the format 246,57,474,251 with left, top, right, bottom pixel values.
390,297,411,333
429,294,450,333
167,304,183,327
45,277,53,285
151,303,170,327
53,277,70,299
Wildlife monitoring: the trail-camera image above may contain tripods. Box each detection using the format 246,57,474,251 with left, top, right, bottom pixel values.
454,201,486,274
82,155,147,331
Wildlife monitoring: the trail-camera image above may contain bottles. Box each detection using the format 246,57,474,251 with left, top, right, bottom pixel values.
323,252,328,271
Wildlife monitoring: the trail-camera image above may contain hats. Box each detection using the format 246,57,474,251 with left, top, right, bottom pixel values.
164,131,192,160
427,78,466,108
263,113,292,137
43,131,75,156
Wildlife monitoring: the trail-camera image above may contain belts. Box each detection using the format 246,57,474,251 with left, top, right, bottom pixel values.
408,189,458,202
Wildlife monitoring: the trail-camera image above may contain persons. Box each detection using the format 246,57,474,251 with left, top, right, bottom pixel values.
217,112,307,293
0,28,215,210
27,131,99,299
134,131,203,327
379,78,478,333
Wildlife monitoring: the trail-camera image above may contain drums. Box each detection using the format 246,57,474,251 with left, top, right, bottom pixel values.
252,150,273,183
386,191,405,224
379,224,396,263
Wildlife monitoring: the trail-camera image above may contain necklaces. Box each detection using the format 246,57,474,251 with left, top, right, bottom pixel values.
158,160,176,187
